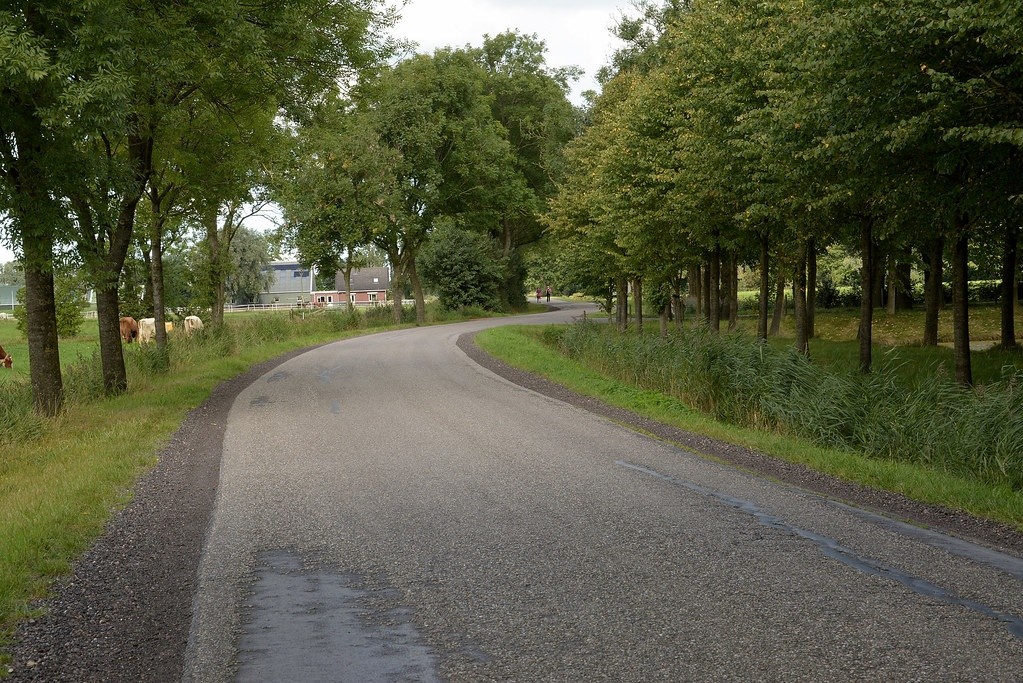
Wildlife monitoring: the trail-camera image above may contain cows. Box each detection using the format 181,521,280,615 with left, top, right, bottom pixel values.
119,316,204,349
0,345,13,369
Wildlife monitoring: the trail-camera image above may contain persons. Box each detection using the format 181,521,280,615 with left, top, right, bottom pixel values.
546,286,551,302
536,287,542,302
